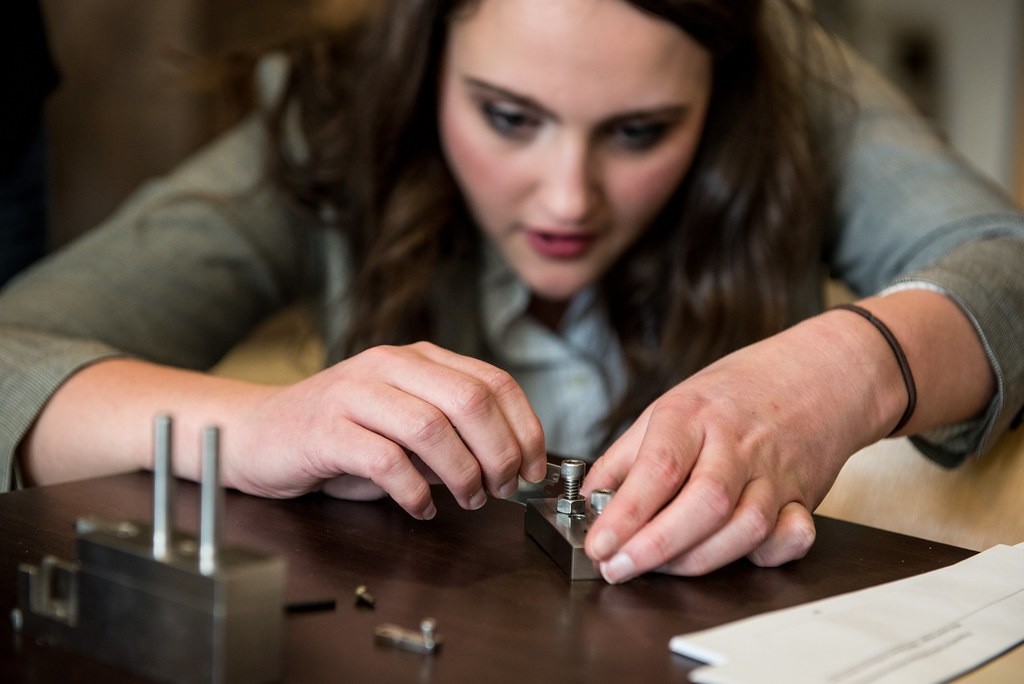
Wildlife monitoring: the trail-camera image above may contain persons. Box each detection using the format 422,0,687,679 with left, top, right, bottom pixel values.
0,0,1024,584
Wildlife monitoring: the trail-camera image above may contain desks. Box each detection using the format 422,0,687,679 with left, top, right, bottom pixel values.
0,470,1023,684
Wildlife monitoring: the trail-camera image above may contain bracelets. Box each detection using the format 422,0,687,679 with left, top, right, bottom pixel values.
832,304,916,440
